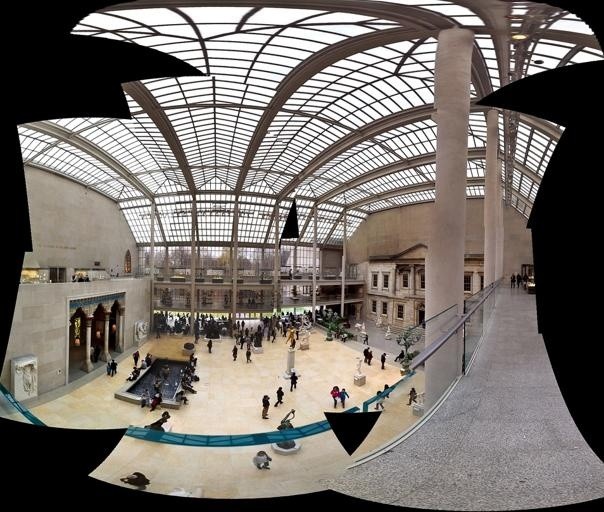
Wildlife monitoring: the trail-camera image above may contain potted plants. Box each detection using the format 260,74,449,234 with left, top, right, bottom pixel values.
326,325,333,341
182,342,196,356
252,336,263,354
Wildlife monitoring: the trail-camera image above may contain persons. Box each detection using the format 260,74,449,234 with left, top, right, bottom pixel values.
289,372,298,392
262,395,270,419
384,384,389,398
140,389,162,411
175,366,199,405
127,351,152,381
363,334,368,345
107,359,117,377
254,450,271,469
274,387,284,407
363,347,373,365
381,353,386,369
394,350,405,362
120,472,150,486
374,391,384,410
407,388,416,405
190,353,197,367
330,385,349,408
154,309,318,363
